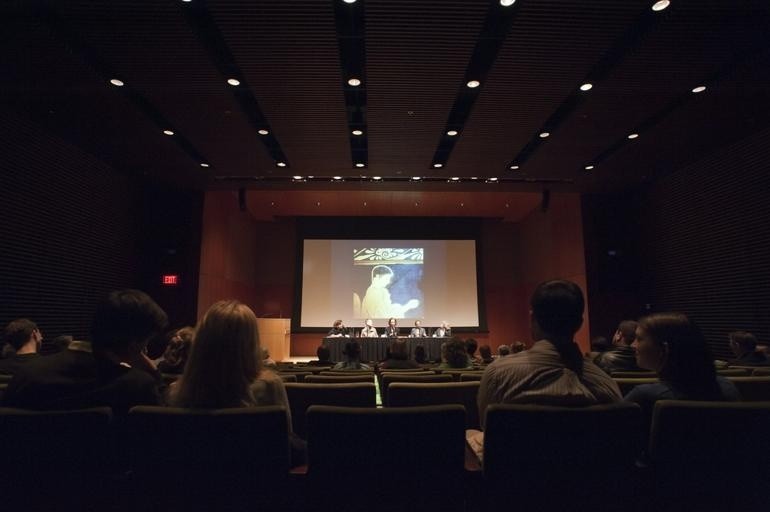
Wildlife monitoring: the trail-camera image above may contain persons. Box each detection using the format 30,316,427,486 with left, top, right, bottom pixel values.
334,339,370,370
5,289,168,407
360,319,379,337
385,318,399,337
592,321,651,373
414,345,430,363
724,332,769,371
382,342,418,370
1,318,74,374
411,320,426,337
467,278,624,464
432,321,451,338
307,344,337,367
159,326,196,384
435,337,524,369
361,265,419,318
163,301,293,432
327,320,350,338
625,315,745,405
262,348,276,366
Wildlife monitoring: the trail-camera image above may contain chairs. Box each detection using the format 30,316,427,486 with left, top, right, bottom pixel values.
0,351,770,511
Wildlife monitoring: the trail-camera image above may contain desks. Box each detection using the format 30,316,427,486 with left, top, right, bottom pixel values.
322,334,456,364
